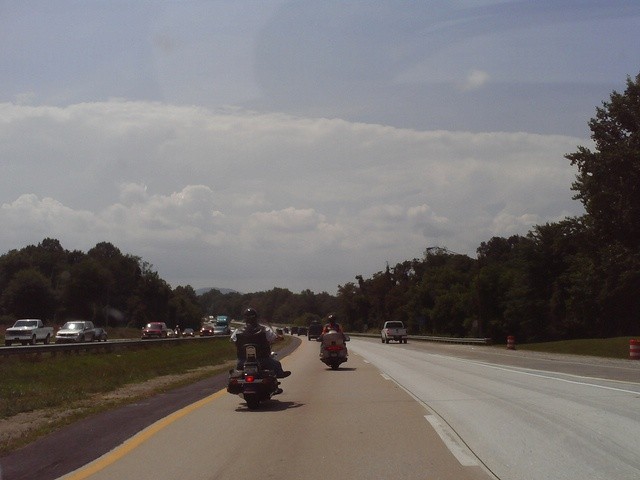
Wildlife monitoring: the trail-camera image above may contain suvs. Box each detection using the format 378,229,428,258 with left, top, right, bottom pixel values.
200,325,213,337
142,322,167,338
308,321,324,341
55,321,94,343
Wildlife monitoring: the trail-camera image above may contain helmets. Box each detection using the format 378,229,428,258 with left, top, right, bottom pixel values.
243,308,257,320
328,315,337,322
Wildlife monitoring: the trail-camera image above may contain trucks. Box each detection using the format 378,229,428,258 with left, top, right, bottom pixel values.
214,316,231,335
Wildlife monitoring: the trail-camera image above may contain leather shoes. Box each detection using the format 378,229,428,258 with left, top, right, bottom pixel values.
277,371,291,378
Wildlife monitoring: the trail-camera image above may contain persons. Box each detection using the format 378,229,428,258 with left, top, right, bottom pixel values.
231,307,293,379
315,314,353,359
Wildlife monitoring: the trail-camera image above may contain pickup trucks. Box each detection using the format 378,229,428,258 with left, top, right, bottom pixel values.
381,320,408,344
5,319,54,345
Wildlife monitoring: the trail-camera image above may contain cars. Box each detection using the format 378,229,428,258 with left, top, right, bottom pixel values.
93,327,109,342
183,328,193,336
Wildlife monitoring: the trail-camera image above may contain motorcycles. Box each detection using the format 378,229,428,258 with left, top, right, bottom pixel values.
316,333,350,370
227,343,283,410
